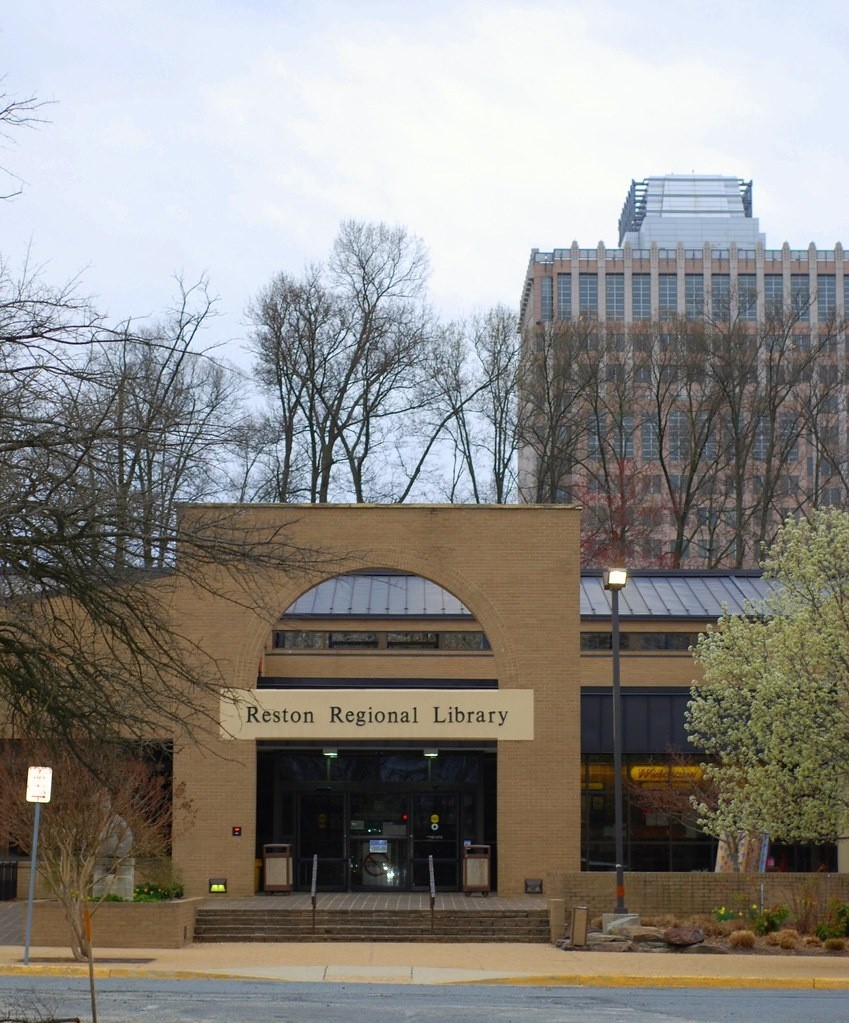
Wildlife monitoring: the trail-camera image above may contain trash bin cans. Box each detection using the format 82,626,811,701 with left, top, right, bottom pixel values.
462,844,491,897
262,843,293,896
570,906,588,947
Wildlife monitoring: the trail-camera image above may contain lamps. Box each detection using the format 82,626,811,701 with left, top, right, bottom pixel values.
525,879,543,894
208,879,226,893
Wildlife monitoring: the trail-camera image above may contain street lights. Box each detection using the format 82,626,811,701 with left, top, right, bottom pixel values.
600,566,629,914
